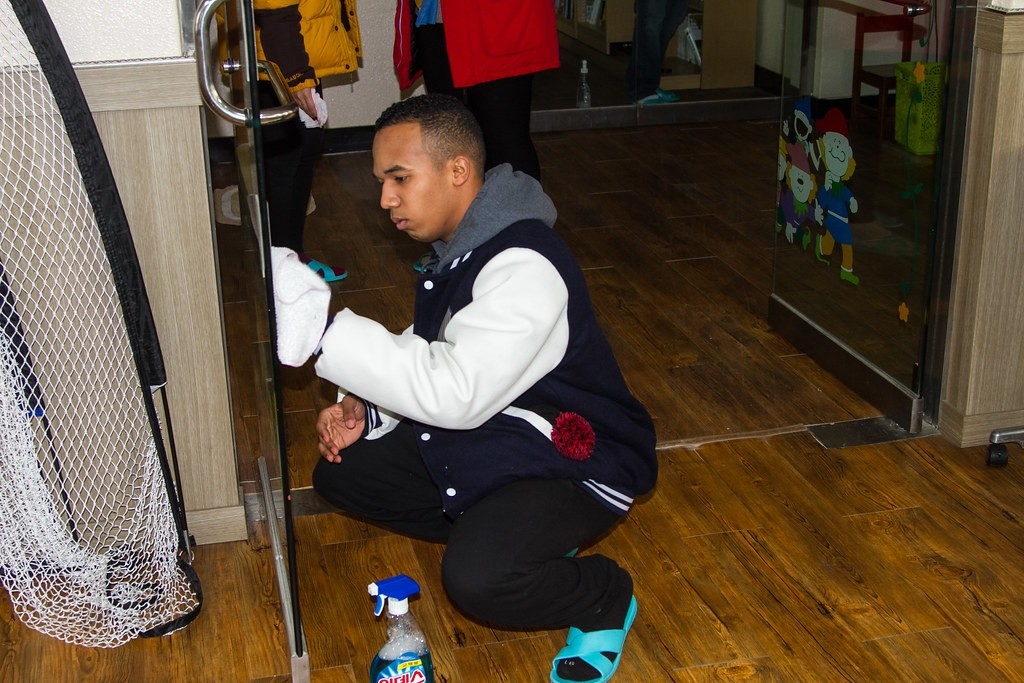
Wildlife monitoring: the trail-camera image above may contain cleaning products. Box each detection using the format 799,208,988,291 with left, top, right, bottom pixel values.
368,573,435,683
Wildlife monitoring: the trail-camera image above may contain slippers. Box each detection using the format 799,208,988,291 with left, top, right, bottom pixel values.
549,594,638,683
413,252,434,271
306,258,349,282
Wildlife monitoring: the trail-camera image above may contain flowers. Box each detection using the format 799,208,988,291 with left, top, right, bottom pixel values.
548,409,596,462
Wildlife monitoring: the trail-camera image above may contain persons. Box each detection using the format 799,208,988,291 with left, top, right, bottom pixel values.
392,0,559,186
215,0,364,283
313,95,658,683
629,0,699,104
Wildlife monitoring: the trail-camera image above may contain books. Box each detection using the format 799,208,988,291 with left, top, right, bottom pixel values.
556,0,604,25
676,12,701,68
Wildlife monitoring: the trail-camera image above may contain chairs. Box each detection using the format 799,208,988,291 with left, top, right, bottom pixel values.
852,9,911,143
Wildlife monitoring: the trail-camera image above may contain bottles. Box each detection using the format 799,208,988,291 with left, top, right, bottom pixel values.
577,60,592,107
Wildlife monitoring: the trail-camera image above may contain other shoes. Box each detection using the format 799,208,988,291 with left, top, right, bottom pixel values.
634,96,667,104
656,88,681,101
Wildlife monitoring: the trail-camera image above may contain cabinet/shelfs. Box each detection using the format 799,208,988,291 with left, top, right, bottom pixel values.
554,0,758,92
